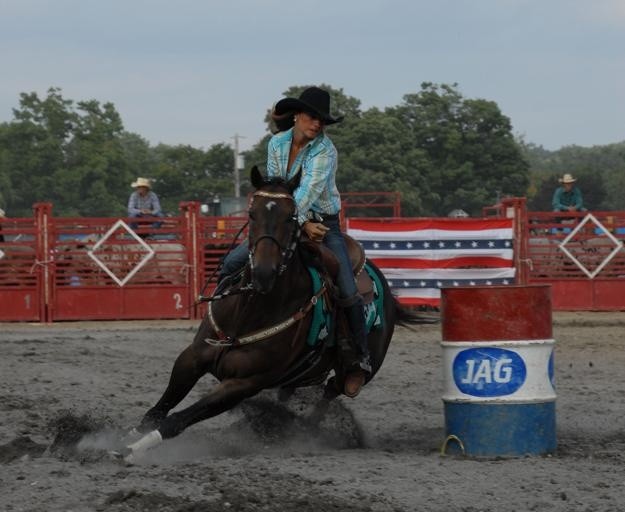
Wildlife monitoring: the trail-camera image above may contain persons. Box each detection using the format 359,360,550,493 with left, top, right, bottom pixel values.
551,174,595,236
217,86,370,398
126,176,163,236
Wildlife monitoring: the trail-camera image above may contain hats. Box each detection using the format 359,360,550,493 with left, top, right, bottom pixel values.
274,87,344,136
557,174,577,184
131,177,152,189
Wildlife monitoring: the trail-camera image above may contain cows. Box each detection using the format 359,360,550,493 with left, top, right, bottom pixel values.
527,237,625,278
58,236,188,285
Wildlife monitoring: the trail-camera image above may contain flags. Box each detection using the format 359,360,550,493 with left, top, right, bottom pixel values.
347,216,517,307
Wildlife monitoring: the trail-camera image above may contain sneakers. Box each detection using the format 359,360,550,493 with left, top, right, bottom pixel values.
343,348,366,396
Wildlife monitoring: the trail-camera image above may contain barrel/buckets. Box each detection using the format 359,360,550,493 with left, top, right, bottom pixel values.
439,283,558,459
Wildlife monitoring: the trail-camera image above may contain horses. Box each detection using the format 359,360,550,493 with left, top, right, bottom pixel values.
119,164,439,468
